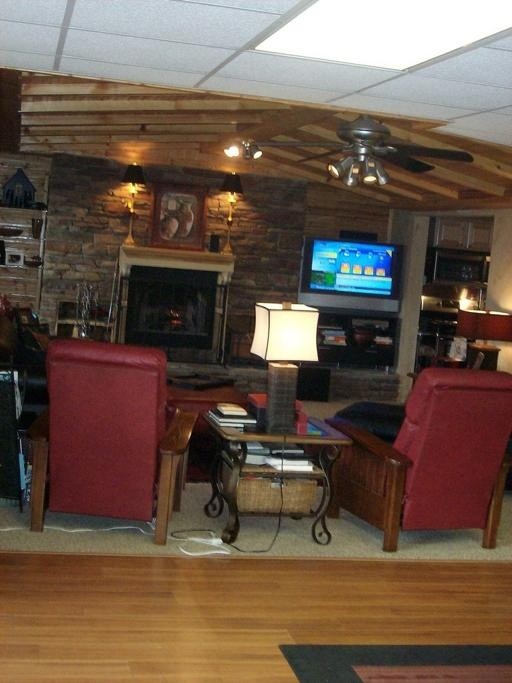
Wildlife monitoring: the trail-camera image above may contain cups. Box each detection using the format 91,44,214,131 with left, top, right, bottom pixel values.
32,218,44,238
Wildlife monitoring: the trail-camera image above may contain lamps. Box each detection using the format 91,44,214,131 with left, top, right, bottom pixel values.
219,171,243,256
121,161,145,246
455,308,512,370
249,301,320,435
222,140,263,160
328,150,397,186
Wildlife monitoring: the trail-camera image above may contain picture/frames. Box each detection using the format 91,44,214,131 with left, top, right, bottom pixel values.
6,251,24,266
150,180,209,252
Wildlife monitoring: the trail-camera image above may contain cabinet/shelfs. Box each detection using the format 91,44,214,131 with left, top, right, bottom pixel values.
55,301,114,343
318,308,400,369
428,216,493,252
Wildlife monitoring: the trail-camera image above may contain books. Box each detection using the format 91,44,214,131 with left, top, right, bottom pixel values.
207,402,257,428
228,442,314,471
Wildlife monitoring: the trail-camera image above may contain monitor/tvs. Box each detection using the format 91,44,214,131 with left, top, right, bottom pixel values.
296,235,406,312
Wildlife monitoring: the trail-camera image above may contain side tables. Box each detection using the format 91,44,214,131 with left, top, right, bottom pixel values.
201,411,352,545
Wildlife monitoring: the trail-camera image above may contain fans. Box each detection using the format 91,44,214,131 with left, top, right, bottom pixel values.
257,115,472,173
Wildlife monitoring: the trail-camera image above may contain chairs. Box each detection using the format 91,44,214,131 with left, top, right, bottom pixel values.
329,369,512,552
26,339,200,545
0,372,27,514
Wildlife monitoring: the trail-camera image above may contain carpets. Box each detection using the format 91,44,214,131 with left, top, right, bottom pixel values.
0,401,512,560
279,643,512,683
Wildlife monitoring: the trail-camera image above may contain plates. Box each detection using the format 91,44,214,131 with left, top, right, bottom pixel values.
0,228,23,236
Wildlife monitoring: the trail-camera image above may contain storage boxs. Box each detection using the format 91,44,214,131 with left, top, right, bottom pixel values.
249,393,302,422
